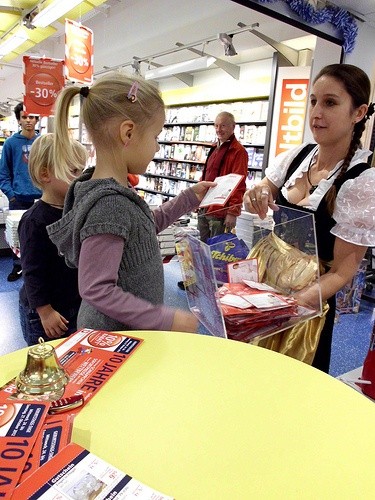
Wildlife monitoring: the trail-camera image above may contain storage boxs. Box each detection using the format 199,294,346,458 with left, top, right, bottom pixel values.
173,202,323,338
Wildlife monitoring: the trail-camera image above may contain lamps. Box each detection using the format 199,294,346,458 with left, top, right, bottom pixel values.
32,1,88,28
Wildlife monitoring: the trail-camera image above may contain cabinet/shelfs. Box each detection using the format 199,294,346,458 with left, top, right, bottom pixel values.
0,95,270,205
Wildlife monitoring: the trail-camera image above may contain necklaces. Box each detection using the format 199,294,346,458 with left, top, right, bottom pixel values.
307,148,345,195
50,204,64,208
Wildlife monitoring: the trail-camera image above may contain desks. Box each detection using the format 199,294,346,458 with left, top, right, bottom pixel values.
0,331,373,499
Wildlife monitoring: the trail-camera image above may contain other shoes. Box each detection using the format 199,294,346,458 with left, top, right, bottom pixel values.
7,264,22,282
178,281,185,290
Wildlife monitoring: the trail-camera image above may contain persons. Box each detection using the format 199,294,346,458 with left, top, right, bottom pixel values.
178,112,249,291
0,103,44,282
18,132,87,346
45,72,217,332
